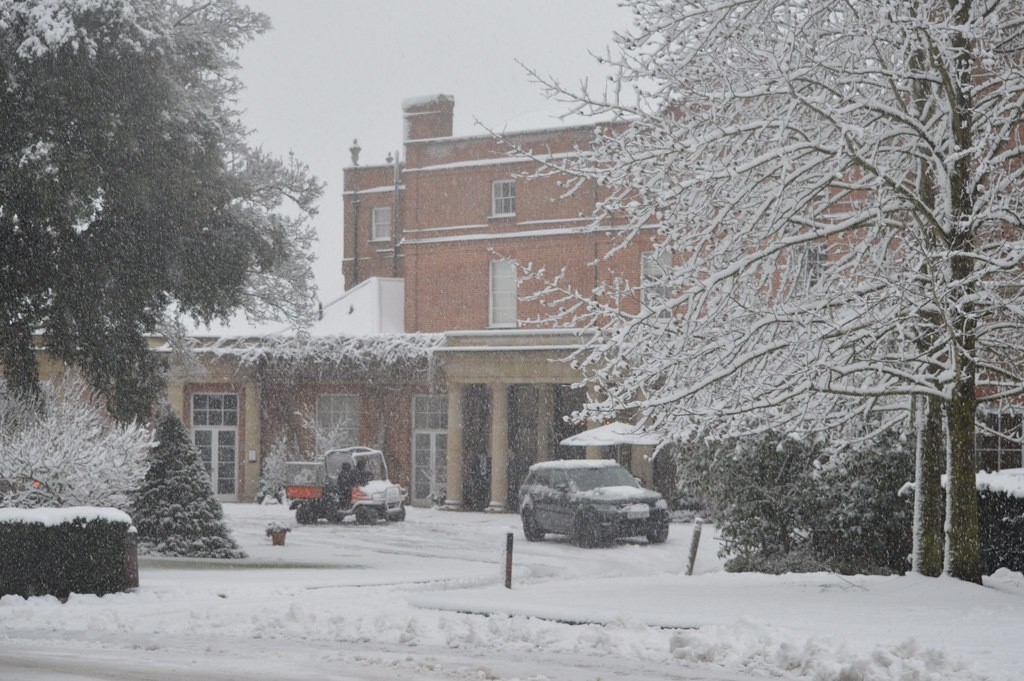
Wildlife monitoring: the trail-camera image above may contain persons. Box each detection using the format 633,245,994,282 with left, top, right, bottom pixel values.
470,446,523,512
337,459,372,505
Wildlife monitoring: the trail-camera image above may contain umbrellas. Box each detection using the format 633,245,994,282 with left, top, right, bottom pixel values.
559,421,662,463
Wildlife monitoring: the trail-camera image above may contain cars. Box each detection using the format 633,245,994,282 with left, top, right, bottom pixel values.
519,457,670,548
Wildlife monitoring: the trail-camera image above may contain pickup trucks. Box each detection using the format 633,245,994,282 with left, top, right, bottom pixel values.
283,445,405,525
